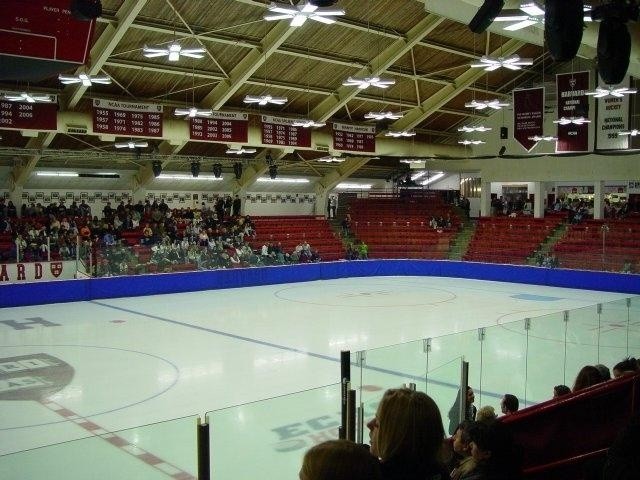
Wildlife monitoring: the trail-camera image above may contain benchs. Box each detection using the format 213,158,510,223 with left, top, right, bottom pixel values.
0,197,638,278
481,368,639,475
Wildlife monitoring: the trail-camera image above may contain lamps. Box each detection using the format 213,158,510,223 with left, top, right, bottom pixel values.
115,141,148,149
364,111,403,120
58,71,112,86
243,95,288,106
317,156,345,163
385,130,417,138
261,0,346,28
400,159,423,163
4,93,52,103
225,148,256,155
143,42,206,61
456,0,640,146
174,108,212,117
342,76,395,89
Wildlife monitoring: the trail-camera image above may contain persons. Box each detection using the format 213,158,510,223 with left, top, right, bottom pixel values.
345,242,357,261
1,193,321,278
553,355,640,399
446,386,477,435
499,393,521,415
294,440,385,480
453,191,458,207
328,195,339,219
490,194,514,214
345,214,353,229
427,214,454,228
469,420,519,479
342,217,350,232
369,387,452,480
450,419,479,480
477,405,497,418
358,241,371,260
553,195,616,220
